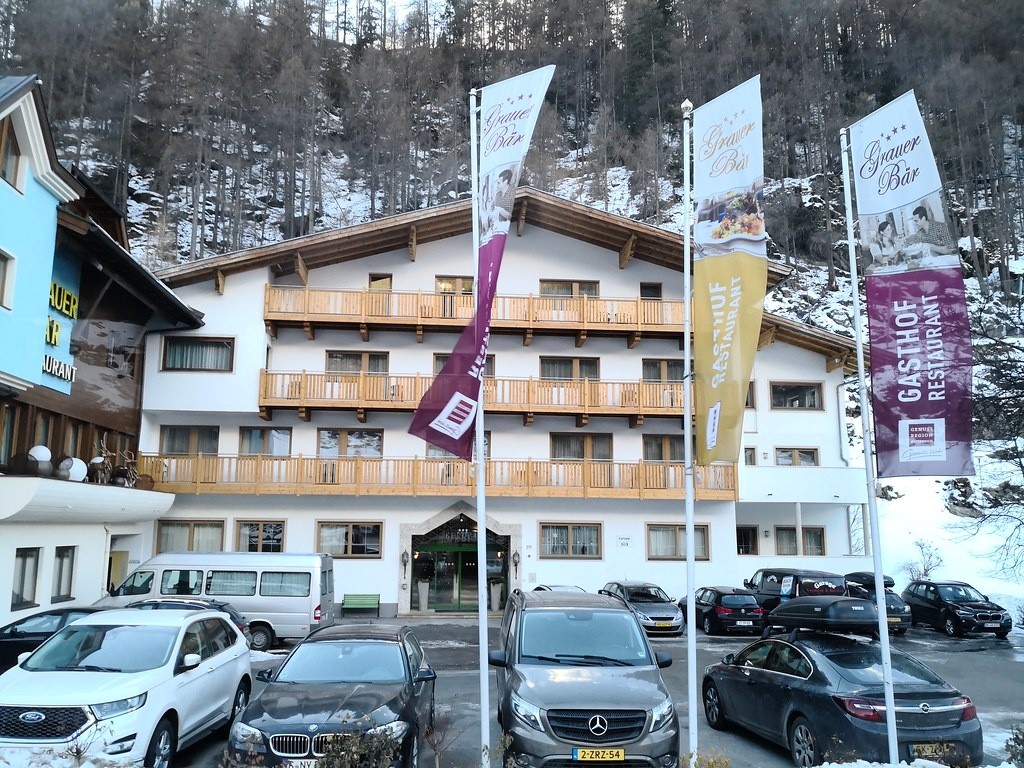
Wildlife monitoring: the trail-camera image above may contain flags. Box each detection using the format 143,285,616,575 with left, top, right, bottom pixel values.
846,90,976,479
691,71,763,502
409,64,558,464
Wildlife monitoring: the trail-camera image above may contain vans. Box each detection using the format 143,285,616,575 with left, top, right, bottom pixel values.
90,549,335,651
742,567,848,622
487,586,681,768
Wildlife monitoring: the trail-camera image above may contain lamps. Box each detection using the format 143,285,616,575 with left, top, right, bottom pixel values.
402,550,409,565
513,550,520,566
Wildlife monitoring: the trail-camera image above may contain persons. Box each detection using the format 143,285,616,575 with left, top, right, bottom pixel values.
869,221,904,267
494,169,515,221
911,206,952,260
480,184,493,232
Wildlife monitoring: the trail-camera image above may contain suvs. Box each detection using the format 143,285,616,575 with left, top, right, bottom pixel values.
227,617,439,768
1,608,255,768
122,594,254,650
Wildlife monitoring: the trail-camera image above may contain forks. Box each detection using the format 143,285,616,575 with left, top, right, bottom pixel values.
694,242,707,258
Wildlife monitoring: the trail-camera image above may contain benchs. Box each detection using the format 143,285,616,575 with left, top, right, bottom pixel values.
341,594,380,618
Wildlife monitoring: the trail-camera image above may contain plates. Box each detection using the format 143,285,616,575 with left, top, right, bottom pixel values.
701,224,765,244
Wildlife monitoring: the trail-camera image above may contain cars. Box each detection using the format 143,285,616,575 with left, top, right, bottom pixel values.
601,579,685,637
677,585,764,635
534,584,588,593
703,595,986,768
900,580,1012,640
843,570,913,636
0,605,125,683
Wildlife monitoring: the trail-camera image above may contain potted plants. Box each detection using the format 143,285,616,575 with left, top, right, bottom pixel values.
416,576,431,611
487,576,505,611
